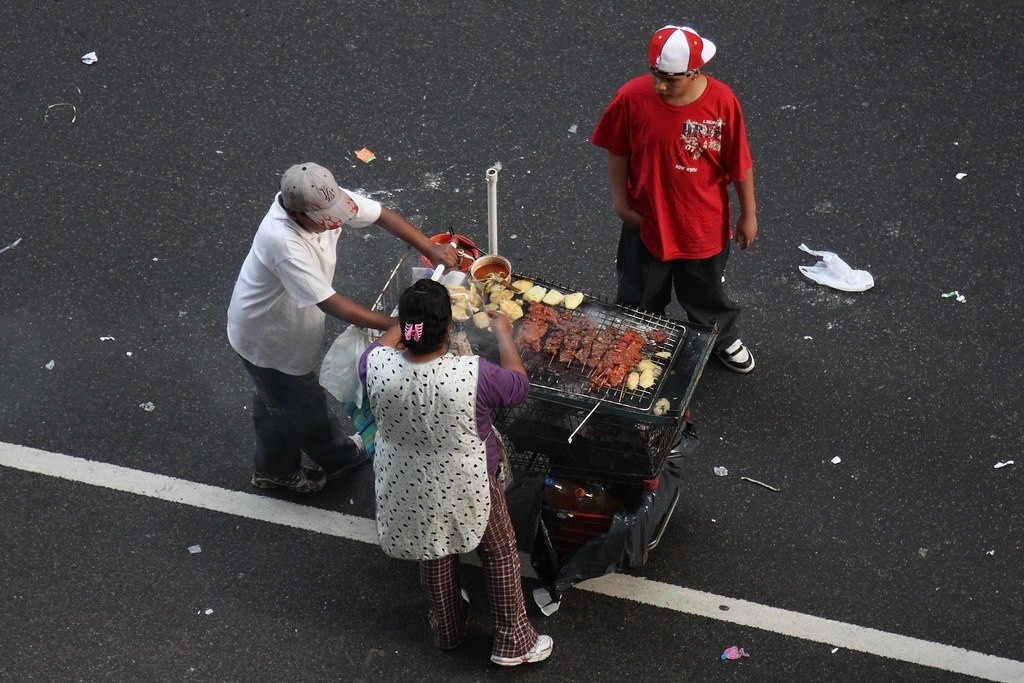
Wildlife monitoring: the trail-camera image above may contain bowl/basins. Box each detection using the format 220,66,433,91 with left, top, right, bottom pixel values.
470,255,511,290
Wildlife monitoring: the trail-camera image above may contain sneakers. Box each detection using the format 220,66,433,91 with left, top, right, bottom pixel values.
459,589,469,604
711,338,755,373
490,635,554,666
318,431,375,480
250,465,327,492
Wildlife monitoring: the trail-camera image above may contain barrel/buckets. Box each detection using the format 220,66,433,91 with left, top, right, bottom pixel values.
421,233,478,274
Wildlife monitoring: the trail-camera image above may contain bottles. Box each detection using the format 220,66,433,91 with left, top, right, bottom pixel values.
575,487,621,517
544,477,575,511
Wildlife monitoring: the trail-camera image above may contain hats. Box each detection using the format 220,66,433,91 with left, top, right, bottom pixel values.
647,25,717,76
280,162,359,230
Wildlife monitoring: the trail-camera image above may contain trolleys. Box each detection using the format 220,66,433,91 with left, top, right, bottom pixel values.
337,236,722,584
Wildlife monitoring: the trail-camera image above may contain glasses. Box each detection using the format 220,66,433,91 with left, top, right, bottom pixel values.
648,65,679,82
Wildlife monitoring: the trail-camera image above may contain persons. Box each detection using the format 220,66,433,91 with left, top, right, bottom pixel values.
590,24,757,373
227,162,459,491
358,278,553,667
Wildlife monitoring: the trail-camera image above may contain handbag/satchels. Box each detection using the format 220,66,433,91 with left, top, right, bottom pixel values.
796,243,874,292
